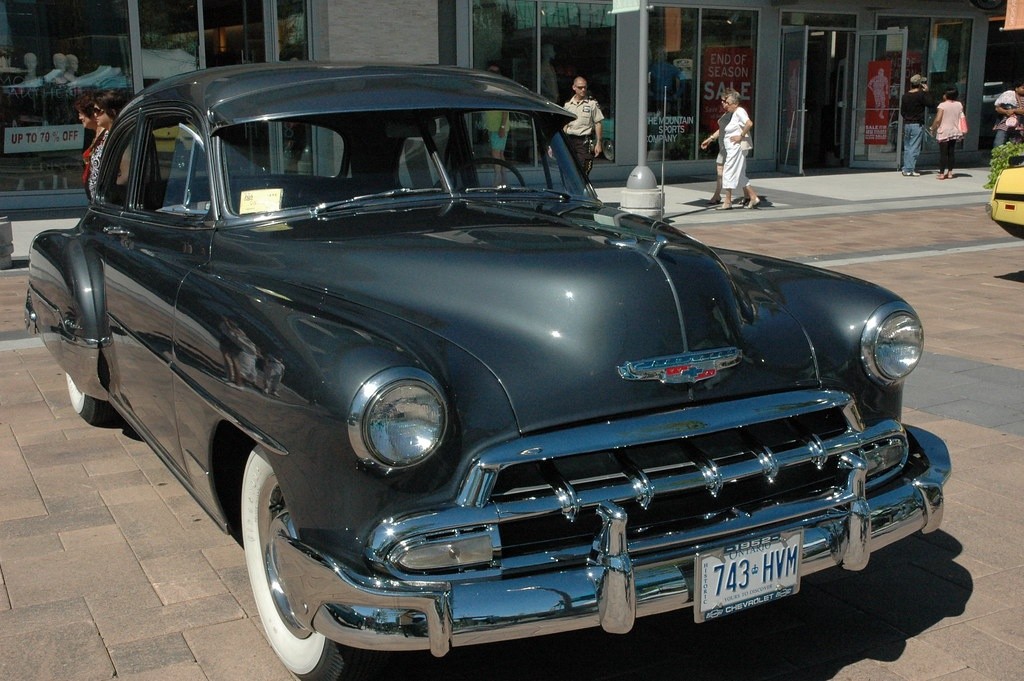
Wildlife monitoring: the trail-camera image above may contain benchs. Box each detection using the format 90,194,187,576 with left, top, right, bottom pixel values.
145,174,330,210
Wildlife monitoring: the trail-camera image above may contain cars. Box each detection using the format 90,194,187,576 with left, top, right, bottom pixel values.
984,153,1024,240
24,60,954,681
594,115,617,165
980,81,1004,105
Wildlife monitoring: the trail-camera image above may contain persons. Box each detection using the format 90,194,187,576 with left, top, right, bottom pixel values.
992,81,1024,147
10,52,78,190
700,87,762,211
649,45,684,150
88,90,132,204
928,85,970,180
899,74,936,176
530,44,558,102
548,76,605,194
74,89,104,202
477,65,512,190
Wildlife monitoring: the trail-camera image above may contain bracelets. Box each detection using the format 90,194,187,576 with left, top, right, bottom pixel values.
710,135,715,141
596,140,602,143
929,126,933,131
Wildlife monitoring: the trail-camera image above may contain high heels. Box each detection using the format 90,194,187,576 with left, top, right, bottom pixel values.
706,196,721,204
739,198,750,204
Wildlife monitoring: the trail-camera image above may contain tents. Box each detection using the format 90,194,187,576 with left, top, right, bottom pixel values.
69,65,129,89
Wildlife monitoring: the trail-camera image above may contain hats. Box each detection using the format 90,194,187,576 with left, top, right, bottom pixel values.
910,74,928,83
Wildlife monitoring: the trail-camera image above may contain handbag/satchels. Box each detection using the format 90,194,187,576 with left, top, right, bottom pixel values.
1006,116,1017,126
960,112,968,133
736,109,753,150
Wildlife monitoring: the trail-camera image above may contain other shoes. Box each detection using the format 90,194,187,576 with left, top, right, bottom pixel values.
744,197,760,209
947,174,953,178
716,203,732,210
936,175,945,179
902,170,921,176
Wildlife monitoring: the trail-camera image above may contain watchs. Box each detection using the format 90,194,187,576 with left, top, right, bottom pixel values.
500,125,505,129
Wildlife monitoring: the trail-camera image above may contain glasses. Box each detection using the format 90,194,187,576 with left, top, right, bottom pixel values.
93,108,108,113
577,86,588,89
721,99,726,102
727,102,734,105
